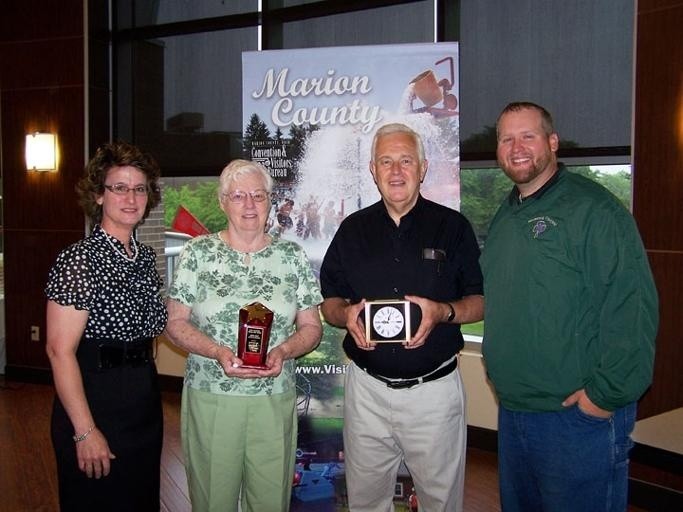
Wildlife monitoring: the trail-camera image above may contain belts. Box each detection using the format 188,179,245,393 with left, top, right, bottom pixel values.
373,356,457,389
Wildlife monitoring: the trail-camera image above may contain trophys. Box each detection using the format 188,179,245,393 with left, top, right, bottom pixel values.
236,302,273,369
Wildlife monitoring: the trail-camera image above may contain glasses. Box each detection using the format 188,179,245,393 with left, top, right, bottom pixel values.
104,184,150,195
222,190,269,201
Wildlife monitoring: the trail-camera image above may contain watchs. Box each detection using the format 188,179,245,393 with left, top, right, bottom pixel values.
446,302,456,326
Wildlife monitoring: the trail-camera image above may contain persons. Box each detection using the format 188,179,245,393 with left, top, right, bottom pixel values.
159,155,323,512
271,188,339,243
39,136,168,511
475,101,663,511
408,486,417,511
316,123,486,511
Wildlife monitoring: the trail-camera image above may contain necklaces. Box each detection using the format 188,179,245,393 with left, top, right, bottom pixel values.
96,225,140,264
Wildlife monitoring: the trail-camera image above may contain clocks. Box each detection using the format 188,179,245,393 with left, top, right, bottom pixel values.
363,299,412,344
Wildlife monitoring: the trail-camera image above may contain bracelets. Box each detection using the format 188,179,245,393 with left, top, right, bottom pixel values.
71,424,96,443
